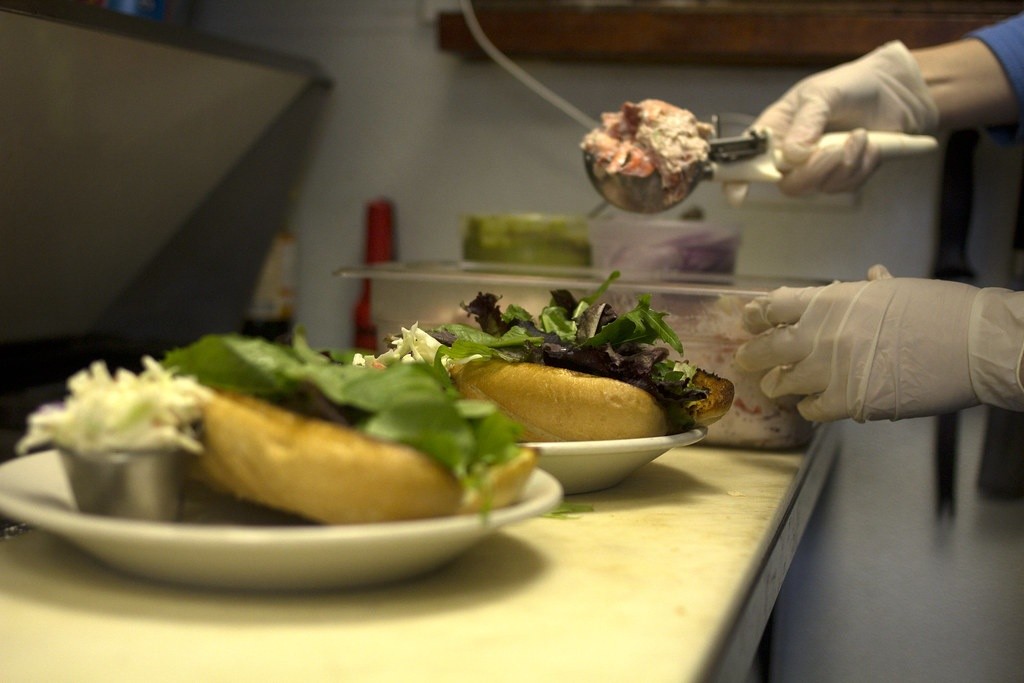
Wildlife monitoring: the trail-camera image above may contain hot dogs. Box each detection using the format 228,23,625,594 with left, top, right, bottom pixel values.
361,270,737,442
145,325,544,523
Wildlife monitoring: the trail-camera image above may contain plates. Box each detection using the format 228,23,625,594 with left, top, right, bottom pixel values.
460,262,599,278
0,447,565,590
514,426,708,494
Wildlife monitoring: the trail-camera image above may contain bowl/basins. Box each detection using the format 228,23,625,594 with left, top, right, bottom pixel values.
586,218,743,283
462,210,592,264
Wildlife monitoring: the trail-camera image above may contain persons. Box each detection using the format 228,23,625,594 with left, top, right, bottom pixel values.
736,12,1024,424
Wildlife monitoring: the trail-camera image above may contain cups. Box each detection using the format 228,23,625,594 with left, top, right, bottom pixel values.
56,442,183,523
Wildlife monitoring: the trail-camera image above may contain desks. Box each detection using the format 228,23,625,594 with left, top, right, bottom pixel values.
0,414,835,683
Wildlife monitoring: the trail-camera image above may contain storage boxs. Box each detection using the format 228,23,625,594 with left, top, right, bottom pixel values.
590,216,744,278
454,209,591,277
331,263,825,450
